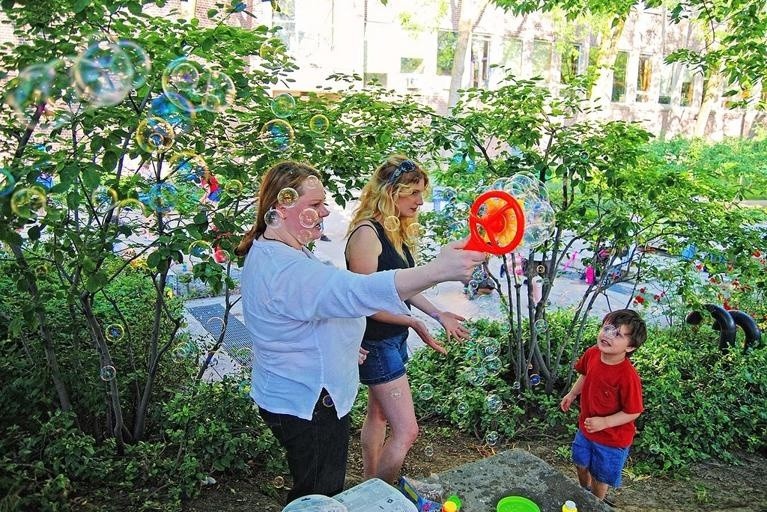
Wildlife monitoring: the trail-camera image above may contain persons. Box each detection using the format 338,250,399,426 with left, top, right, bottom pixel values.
232,161,488,505
196,170,225,227
519,242,558,312
341,152,471,487
561,309,647,504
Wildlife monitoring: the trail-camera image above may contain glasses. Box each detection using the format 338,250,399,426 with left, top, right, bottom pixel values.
389,160,416,183
